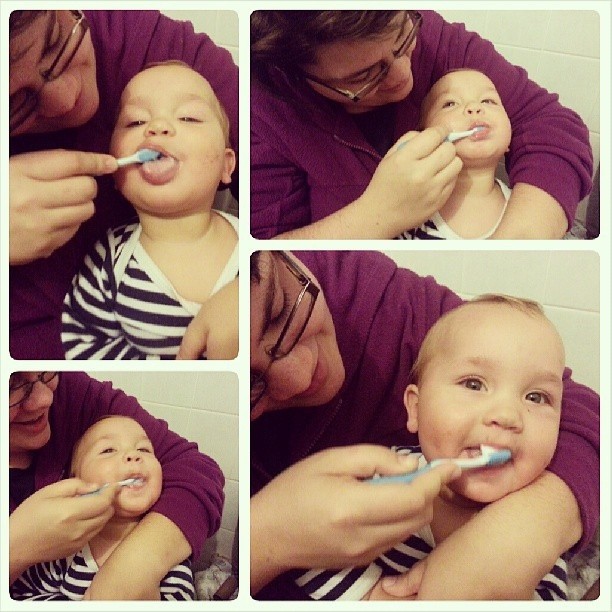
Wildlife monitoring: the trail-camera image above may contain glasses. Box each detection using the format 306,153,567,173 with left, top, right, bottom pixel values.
290,11,423,102
9,371,57,407
250,251,320,409
9,11,89,132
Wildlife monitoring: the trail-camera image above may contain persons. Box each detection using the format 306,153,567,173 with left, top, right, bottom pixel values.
61,60,239,359
250,10,593,240
10,371,225,600
9,10,239,360
394,69,513,240
286,293,566,600
9,415,196,600
250,251,600,599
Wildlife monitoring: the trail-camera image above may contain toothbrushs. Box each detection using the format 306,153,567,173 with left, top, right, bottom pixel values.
396,127,484,153
362,443,512,485
80,478,136,496
117,148,161,168
